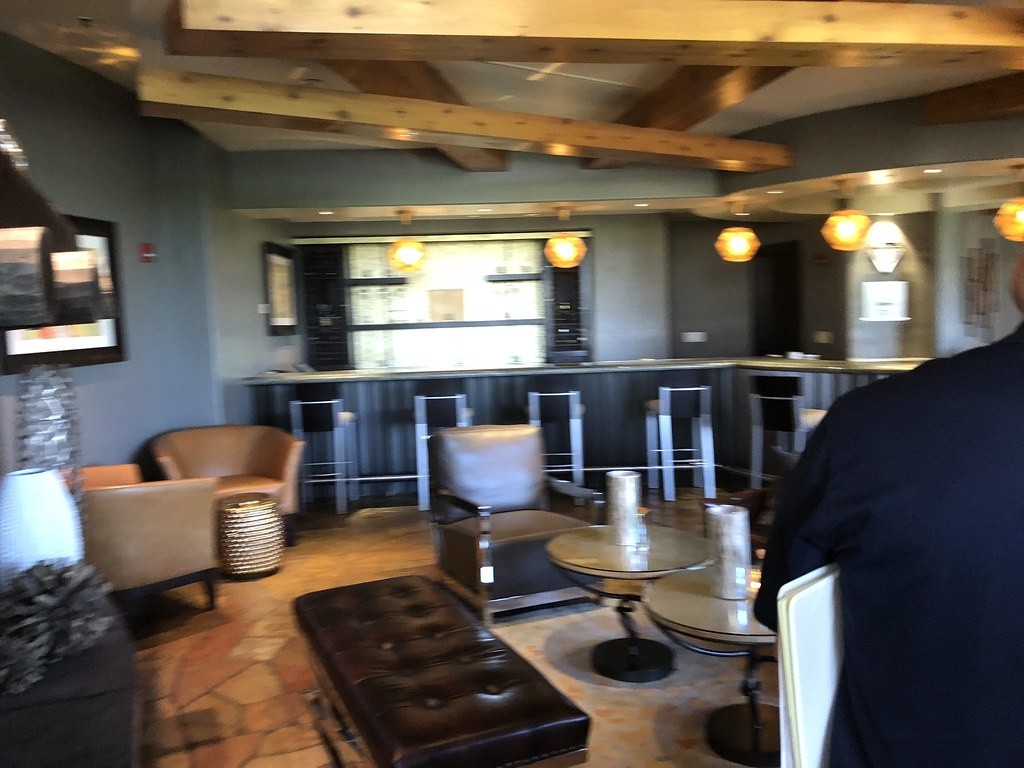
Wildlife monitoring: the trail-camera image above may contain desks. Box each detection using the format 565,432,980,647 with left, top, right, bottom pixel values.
543,523,713,685
644,563,782,768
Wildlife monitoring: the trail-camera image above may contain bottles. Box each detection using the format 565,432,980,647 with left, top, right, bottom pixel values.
754,549,765,568
636,507,648,552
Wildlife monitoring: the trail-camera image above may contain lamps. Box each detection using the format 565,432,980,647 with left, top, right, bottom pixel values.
543,205,588,268
820,178,872,252
388,210,429,273
713,201,762,262
991,164,1024,242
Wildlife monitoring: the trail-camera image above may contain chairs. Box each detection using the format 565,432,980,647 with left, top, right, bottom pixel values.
529,390,586,507
291,398,361,515
749,393,828,489
152,424,305,546
415,393,475,511
66,464,223,609
644,385,718,502
427,423,607,624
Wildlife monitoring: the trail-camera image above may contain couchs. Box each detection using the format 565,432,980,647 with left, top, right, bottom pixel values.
0,597,155,768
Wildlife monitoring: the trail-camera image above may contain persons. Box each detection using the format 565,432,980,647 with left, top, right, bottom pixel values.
751,252,1022,768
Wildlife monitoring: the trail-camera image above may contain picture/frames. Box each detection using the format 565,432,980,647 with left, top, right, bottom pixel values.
0,215,126,377
263,240,299,337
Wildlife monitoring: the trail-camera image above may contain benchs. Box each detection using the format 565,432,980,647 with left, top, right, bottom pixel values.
292,574,593,768
